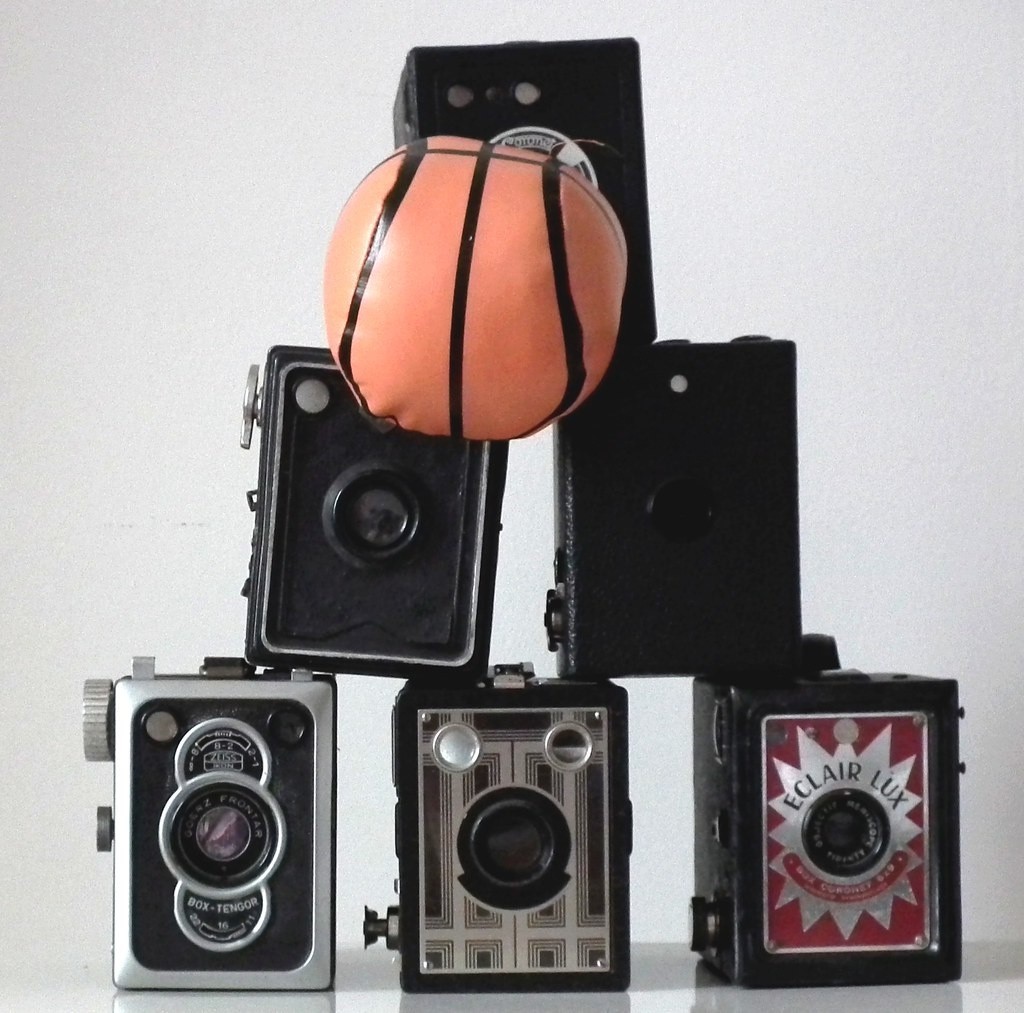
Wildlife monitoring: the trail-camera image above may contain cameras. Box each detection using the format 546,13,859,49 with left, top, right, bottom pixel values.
81,36,965,992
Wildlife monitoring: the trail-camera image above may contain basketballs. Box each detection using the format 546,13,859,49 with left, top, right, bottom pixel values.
320,133,630,443
